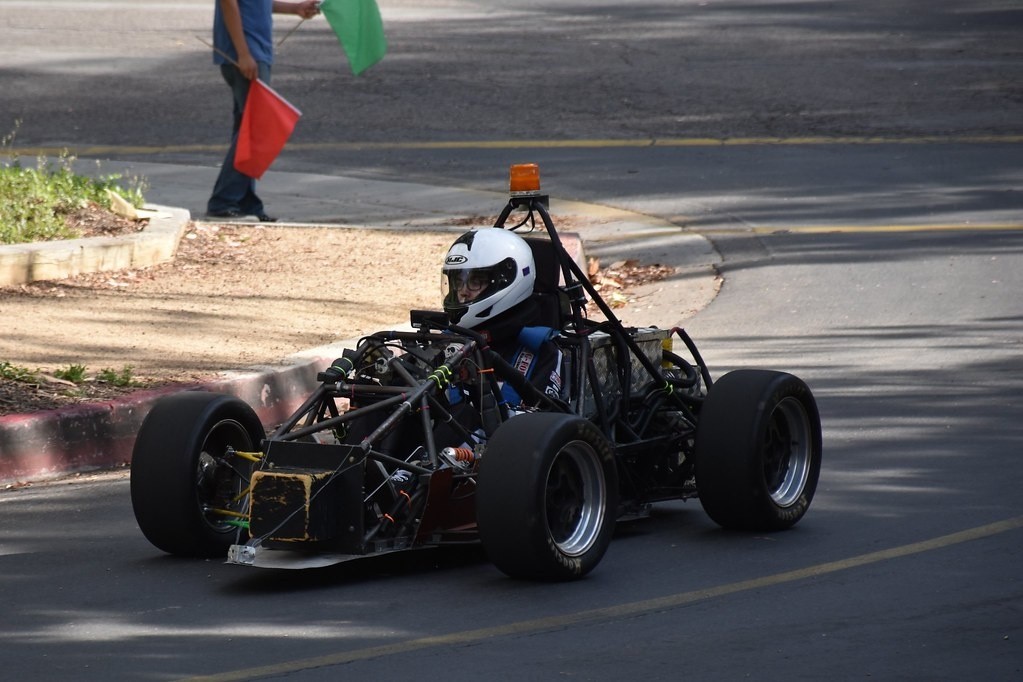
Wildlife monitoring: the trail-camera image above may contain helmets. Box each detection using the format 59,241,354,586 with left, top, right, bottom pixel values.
440,227,536,330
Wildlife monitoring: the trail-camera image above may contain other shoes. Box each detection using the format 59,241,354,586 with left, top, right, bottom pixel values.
206,209,260,222
258,213,280,222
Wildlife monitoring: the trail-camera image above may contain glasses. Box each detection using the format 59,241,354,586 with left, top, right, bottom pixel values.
452,276,494,291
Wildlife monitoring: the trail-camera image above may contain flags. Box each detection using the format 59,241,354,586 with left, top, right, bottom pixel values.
233,80,302,180
311,0,388,75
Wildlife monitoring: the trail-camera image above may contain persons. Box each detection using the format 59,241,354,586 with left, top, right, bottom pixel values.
342,228,565,524
206,0,322,221
